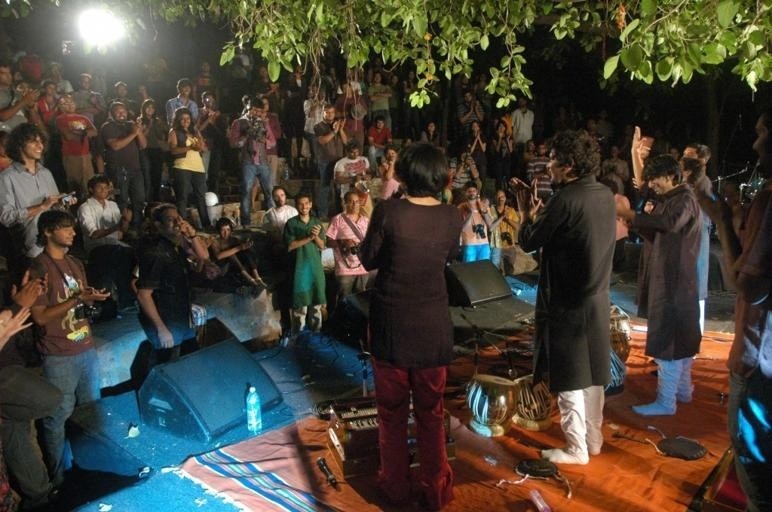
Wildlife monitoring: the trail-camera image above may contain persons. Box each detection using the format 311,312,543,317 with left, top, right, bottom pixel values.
695,106,772,512
517,130,617,465
405,72,562,283
358,144,465,509
1,48,404,363
2,208,112,512
586,115,710,415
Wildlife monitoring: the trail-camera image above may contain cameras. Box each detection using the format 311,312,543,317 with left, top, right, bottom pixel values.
75,306,101,319
501,232,512,244
351,246,361,254
471,224,486,239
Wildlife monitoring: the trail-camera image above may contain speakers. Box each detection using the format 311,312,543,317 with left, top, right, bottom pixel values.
443,259,536,333
343,284,479,349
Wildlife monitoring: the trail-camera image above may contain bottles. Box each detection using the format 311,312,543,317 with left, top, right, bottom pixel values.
244,385,263,432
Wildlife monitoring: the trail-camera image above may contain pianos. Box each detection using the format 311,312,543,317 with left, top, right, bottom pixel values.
326,404,457,479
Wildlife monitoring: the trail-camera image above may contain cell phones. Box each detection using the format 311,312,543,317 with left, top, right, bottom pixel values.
63,194,74,203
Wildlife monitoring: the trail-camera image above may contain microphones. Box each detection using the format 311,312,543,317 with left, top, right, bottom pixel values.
316,457,337,486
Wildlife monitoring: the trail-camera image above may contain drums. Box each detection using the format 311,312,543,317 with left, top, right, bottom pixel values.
468,376,520,439
512,374,553,432
604,348,625,397
610,307,631,363
